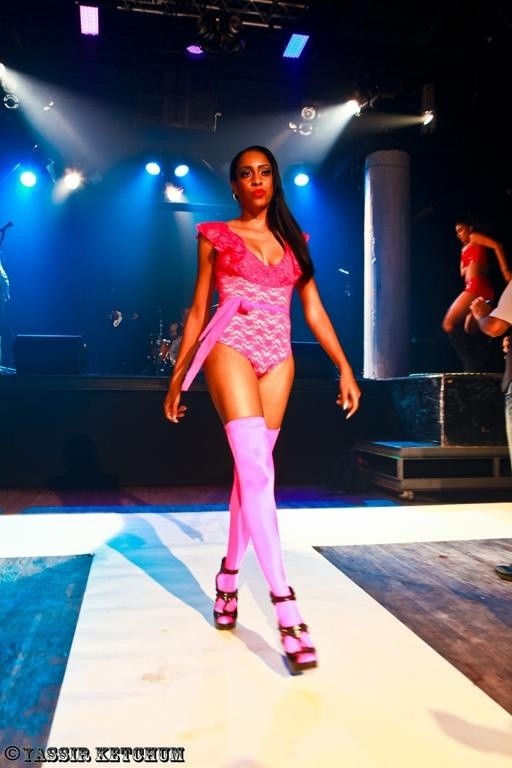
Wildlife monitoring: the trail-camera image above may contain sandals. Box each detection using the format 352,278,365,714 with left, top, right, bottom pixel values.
214,556,239,629
271,586,318,672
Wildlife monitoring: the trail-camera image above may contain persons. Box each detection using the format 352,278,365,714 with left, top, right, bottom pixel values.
162,141,362,676
0,265,11,301
465,277,512,583
440,217,512,374
160,305,192,372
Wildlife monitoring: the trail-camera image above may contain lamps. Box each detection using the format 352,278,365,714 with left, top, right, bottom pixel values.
194,6,247,57
346,89,379,116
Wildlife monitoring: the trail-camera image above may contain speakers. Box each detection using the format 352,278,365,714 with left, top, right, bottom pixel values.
12,334,83,375
291,341,337,378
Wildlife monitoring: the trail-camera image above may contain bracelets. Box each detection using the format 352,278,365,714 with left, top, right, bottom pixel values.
502,268,508,274
